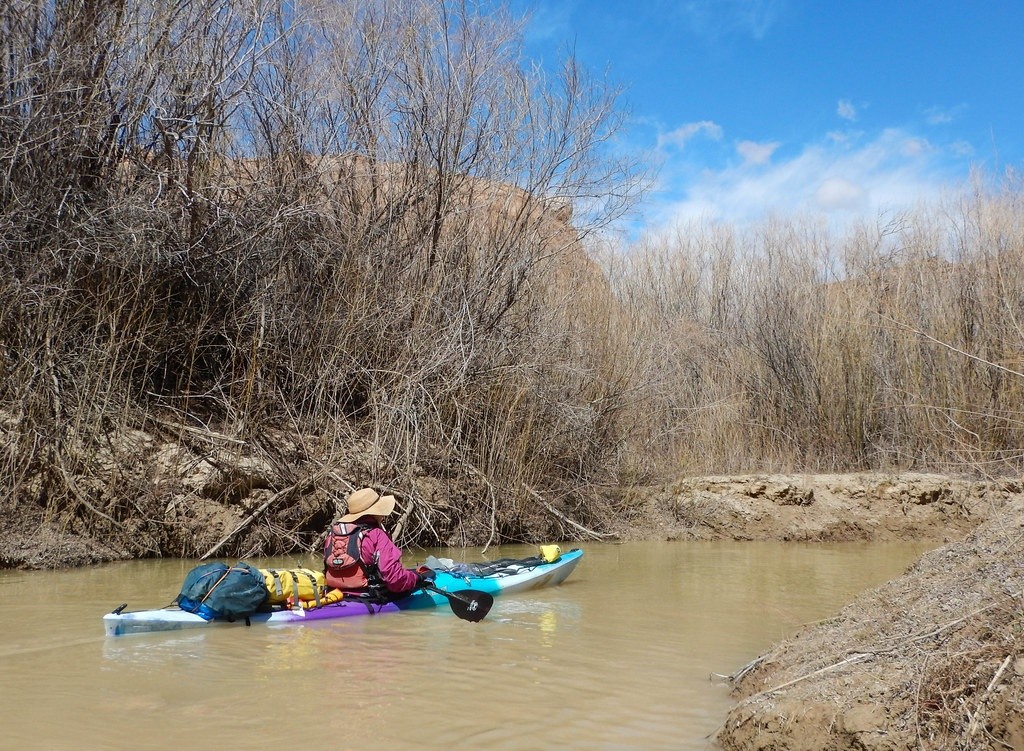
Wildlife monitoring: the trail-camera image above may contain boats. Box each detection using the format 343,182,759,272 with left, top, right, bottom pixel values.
102,545,581,640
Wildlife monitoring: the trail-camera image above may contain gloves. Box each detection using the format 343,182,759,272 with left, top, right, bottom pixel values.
420,569,436,586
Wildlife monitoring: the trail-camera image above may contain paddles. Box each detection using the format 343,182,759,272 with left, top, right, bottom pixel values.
424,585,493,622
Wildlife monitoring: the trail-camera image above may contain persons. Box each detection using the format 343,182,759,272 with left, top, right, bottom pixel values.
325,487,436,603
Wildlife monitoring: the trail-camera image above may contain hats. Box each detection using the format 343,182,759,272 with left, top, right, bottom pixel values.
337,487,395,523
